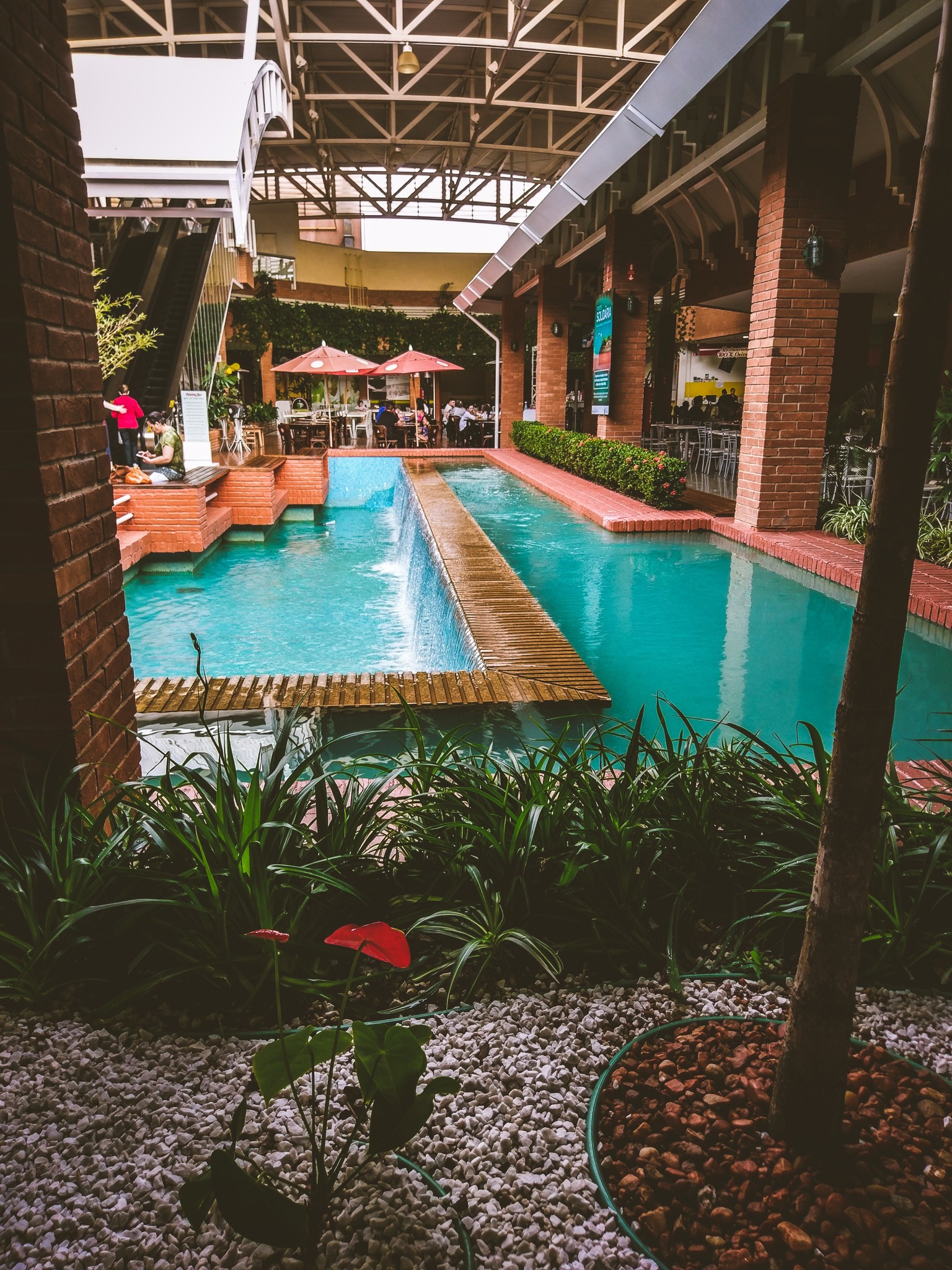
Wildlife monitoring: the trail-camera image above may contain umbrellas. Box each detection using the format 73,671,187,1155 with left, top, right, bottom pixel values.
268,339,465,448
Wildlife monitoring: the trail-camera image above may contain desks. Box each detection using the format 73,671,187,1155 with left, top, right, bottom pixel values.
290,421,329,450
669,425,702,463
346,411,367,447
284,412,311,417
394,425,416,448
704,430,741,474
651,424,678,441
471,419,495,448
242,427,265,447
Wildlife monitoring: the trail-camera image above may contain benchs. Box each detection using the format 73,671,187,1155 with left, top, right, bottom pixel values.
219,455,287,470
293,447,327,456
113,466,230,490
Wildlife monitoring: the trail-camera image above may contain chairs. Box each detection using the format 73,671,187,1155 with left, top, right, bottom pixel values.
823,428,876,504
922,457,952,522
641,417,743,482
243,423,259,449
273,400,493,456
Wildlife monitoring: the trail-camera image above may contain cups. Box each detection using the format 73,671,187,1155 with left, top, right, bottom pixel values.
478,415,481,420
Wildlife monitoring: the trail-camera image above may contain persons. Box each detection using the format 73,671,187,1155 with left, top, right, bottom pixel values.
677,387,742,426
138,411,185,481
354,400,368,411
375,398,501,448
103,384,144,471
346,376,359,404
324,375,344,404
311,374,324,404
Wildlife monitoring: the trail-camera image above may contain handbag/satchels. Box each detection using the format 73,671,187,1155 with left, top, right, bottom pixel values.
110,464,131,484
126,468,152,484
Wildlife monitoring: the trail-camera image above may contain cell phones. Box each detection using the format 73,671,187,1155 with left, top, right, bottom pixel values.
136,454,143,457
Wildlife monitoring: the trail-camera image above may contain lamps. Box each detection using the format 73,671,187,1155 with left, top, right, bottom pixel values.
626,290,635,316
512,340,518,352
553,321,560,336
396,41,420,74
807,224,824,268
